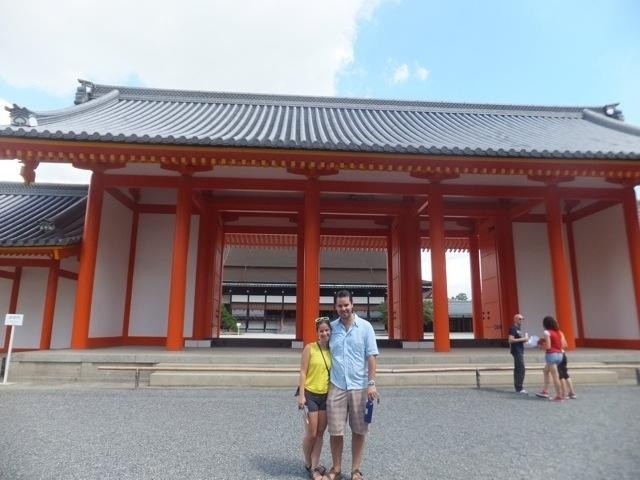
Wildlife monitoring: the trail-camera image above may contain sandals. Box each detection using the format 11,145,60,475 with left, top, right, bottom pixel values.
305,463,364,480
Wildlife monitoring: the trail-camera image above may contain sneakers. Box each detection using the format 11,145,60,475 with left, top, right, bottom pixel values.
536,391,577,401
518,388,527,394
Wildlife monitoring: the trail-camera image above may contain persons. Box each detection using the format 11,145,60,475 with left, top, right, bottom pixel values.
295,315,332,480
321,289,379,480
507,311,531,394
557,329,578,401
534,315,566,404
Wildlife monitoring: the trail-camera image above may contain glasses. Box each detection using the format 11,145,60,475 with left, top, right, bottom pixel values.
517,318,524,320
315,317,330,322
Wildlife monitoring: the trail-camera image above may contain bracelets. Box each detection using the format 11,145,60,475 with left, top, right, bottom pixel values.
365,380,376,386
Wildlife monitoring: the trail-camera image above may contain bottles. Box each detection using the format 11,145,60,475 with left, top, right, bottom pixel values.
301,404,310,425
524,332,529,345
363,395,374,423
